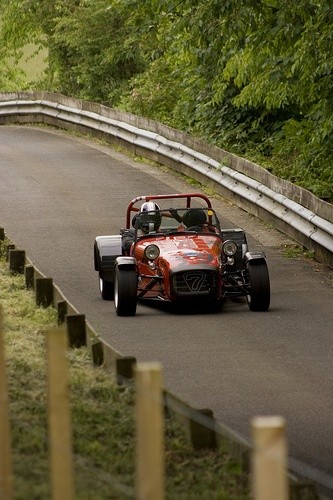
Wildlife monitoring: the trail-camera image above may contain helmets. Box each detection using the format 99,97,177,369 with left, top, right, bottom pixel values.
138,202,161,232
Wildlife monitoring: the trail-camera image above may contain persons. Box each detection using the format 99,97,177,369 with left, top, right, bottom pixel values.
130,201,166,239
179,208,217,234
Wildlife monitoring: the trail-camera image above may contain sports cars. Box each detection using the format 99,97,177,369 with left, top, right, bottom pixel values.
93,193,271,316
